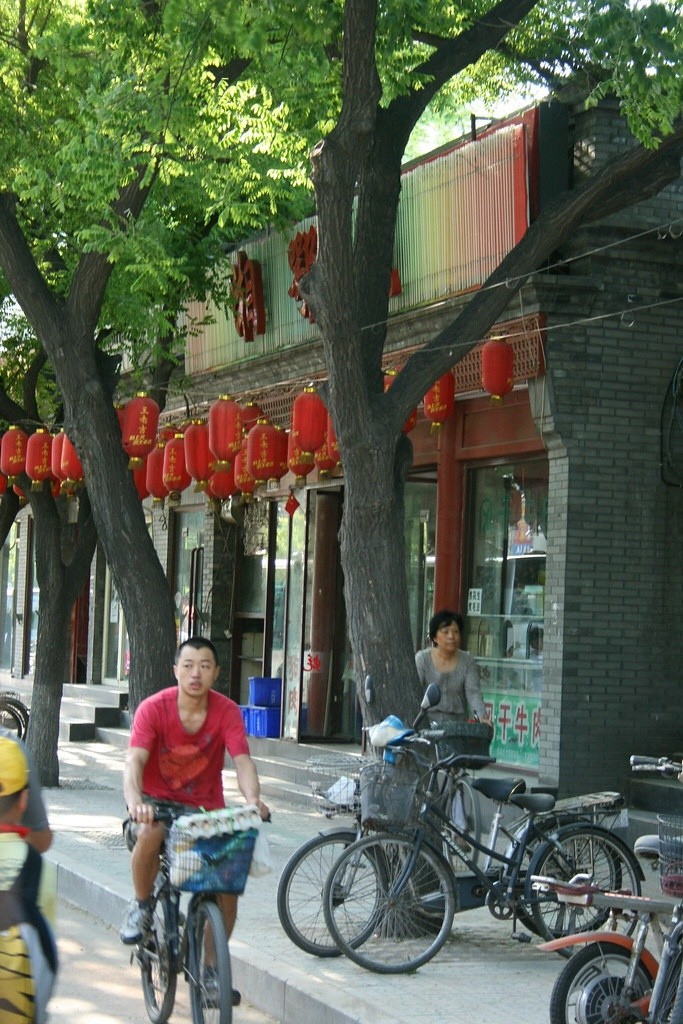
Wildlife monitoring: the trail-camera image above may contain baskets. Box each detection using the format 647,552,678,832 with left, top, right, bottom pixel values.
164,827,257,894
657,814,683,897
360,760,419,830
434,719,494,767
305,754,368,818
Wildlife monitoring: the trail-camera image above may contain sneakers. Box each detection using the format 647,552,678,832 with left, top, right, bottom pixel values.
122,901,151,944
201,979,241,1007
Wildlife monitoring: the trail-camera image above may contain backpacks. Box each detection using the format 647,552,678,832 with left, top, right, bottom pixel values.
0,840,57,1024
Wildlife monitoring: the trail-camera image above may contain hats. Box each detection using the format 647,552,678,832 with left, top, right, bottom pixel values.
0,735,29,796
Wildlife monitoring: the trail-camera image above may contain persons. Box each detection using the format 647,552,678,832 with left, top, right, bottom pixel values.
414,609,494,853
0,727,51,1024
120,636,269,1007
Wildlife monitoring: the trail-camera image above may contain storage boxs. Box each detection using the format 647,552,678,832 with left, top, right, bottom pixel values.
238,677,282,740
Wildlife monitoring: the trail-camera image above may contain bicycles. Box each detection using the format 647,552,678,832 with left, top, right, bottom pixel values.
323,746,647,974
130,804,272,1024
629,756,683,1024
0,702,26,740
0,691,30,742
276,676,623,959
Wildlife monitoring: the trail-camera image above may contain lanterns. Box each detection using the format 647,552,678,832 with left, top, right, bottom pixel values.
0,335,515,509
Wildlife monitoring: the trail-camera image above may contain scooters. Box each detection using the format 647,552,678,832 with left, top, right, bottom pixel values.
529,873,680,1024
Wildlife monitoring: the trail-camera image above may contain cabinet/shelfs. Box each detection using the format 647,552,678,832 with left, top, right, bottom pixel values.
467,555,546,660
